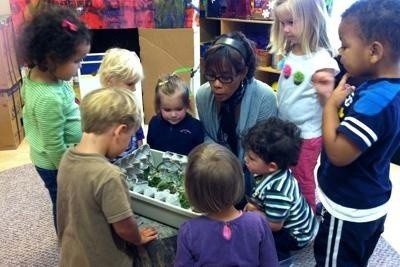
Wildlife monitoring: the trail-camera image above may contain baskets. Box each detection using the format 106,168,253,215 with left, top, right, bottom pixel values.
255,46,273,67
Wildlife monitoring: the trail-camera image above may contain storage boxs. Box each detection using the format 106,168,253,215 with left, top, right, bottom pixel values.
0,13,25,151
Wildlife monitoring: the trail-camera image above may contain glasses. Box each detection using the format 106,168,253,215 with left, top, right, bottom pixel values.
203,69,243,85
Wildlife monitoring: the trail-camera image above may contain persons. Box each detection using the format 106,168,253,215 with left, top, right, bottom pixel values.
98,47,144,165
147,73,204,155
174,142,278,266
266,1,340,213
240,116,319,266
56,86,158,267
195,31,277,164
17,4,91,237
311,1,400,267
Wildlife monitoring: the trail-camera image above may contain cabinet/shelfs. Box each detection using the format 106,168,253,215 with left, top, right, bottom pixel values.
200,15,283,94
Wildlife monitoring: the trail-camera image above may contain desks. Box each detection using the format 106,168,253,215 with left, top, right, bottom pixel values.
127,211,180,267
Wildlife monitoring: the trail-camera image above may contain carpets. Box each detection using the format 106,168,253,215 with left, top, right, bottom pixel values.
0,161,400,267
